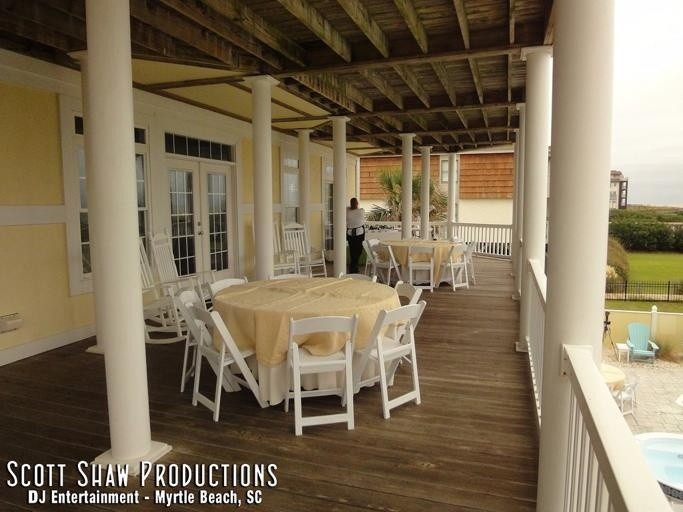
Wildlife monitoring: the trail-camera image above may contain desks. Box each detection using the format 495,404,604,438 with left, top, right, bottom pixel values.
602,364,625,393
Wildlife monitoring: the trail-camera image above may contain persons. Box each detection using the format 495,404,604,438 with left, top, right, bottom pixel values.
345,197,367,273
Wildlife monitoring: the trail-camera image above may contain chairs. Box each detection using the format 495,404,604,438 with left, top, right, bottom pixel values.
140,220,476,437
627,322,659,364
614,383,638,422
